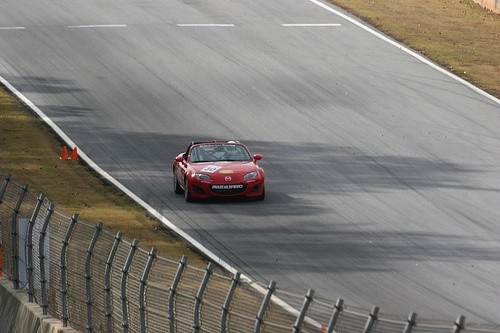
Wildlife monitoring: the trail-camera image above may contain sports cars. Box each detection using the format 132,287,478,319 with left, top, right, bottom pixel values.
173,142,267,204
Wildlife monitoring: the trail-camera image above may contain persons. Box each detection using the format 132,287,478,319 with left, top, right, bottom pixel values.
220,141,244,160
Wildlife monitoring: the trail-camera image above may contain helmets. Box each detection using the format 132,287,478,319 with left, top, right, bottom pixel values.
225,141,238,152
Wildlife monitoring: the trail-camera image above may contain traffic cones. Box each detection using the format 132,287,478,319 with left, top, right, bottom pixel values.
70,147,78,160
60,147,71,160
